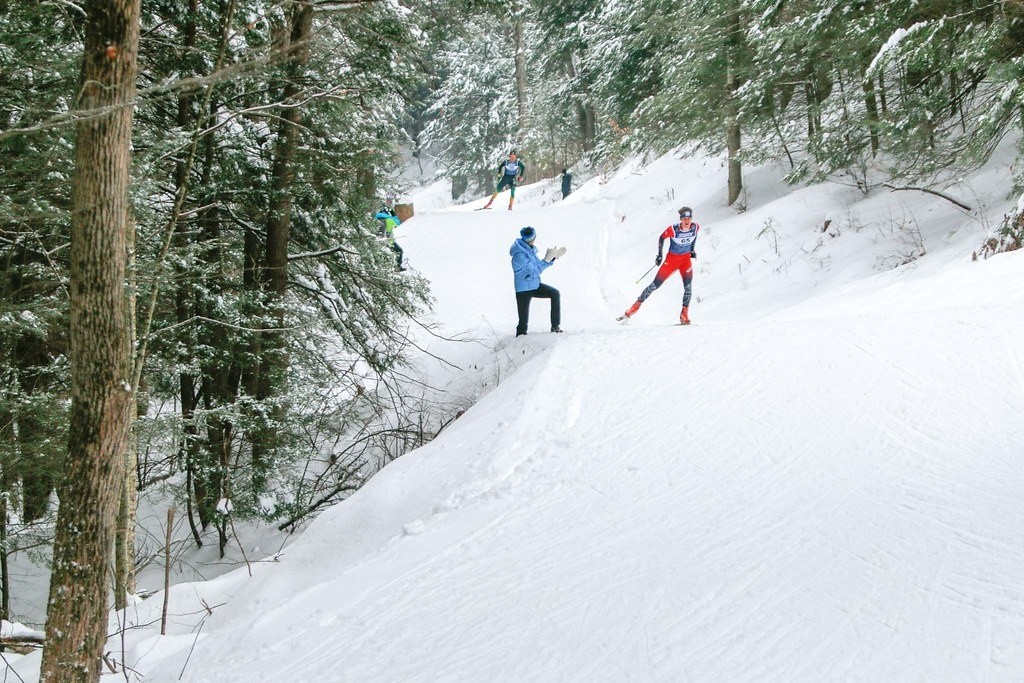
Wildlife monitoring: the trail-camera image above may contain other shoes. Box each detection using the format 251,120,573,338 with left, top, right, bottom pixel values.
509,204,513,210
395,267,406,272
551,325,563,332
484,202,492,208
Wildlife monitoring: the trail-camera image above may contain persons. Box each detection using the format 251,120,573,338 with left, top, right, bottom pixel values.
625,207,699,325
562,168,572,199
483,151,525,210
373,194,407,272
510,226,567,337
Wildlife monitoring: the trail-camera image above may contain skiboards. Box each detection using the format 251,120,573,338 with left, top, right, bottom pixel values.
473,207,492,211
615,312,689,326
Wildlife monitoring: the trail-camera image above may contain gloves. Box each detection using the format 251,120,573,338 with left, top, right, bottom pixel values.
553,246,567,258
690,251,696,258
497,177,500,182
544,248,556,264
655,256,662,266
518,177,522,181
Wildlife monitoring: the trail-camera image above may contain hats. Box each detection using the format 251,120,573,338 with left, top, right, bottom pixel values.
520,226,535,242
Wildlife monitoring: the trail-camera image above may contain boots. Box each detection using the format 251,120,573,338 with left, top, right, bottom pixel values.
625,301,641,316
680,306,690,323
